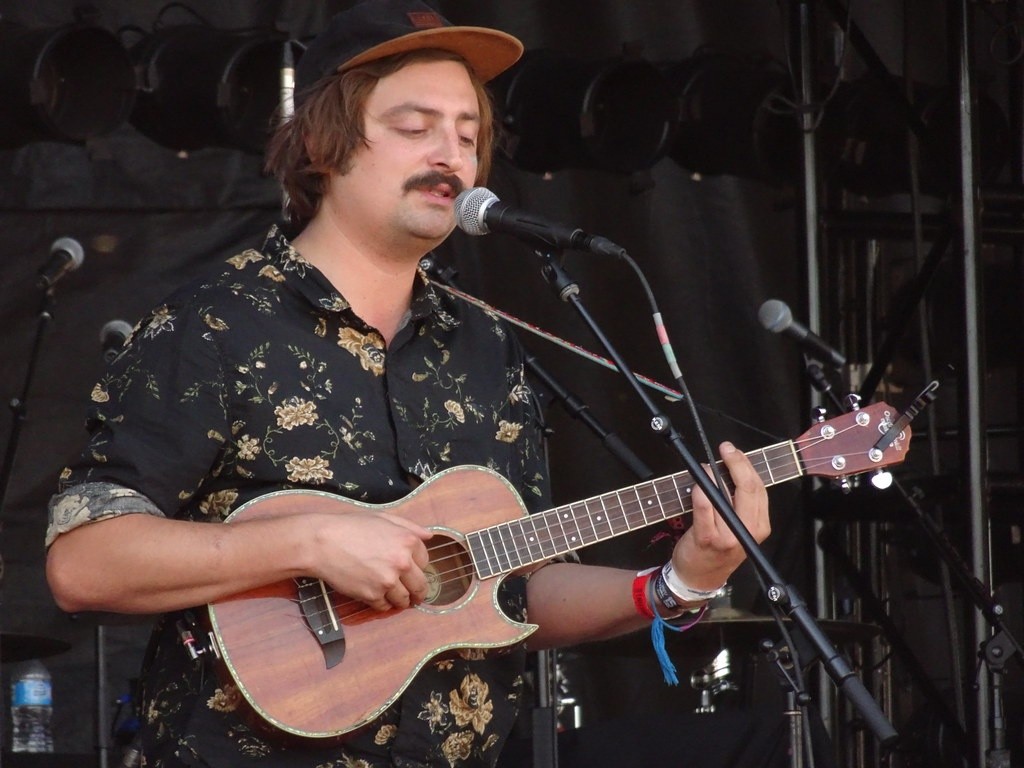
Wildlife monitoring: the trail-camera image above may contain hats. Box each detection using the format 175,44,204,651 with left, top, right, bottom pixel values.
294,0,526,96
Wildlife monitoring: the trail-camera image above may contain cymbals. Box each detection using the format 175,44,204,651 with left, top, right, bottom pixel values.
578,601,885,659
1,631,74,666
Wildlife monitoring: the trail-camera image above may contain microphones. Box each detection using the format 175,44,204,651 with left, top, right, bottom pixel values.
453,187,626,260
35,238,85,286
99,320,134,364
758,298,846,367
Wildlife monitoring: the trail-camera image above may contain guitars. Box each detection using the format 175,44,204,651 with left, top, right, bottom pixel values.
197,376,946,758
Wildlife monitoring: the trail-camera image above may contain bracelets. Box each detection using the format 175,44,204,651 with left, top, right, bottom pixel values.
633,560,727,685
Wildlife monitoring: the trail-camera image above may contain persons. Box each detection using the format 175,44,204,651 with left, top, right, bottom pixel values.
45,0,772,768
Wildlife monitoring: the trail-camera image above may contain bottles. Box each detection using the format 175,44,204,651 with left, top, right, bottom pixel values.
9,657,54,753
